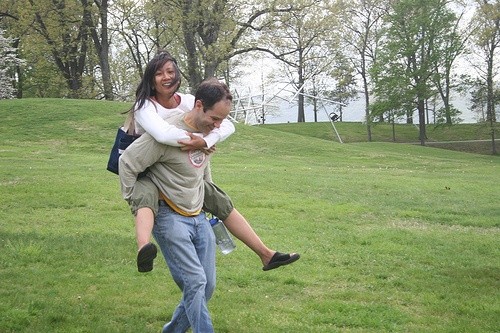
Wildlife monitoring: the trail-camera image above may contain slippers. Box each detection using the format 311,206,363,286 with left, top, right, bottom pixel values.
263,250,300,271
137,242,157,272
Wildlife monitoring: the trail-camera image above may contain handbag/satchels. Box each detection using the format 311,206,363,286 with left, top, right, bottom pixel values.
106,104,140,175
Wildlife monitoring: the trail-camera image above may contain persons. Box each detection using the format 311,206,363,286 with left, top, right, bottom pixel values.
118,50,302,273
117,77,234,332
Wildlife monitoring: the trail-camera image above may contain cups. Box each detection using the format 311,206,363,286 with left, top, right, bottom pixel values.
209,213,234,255
